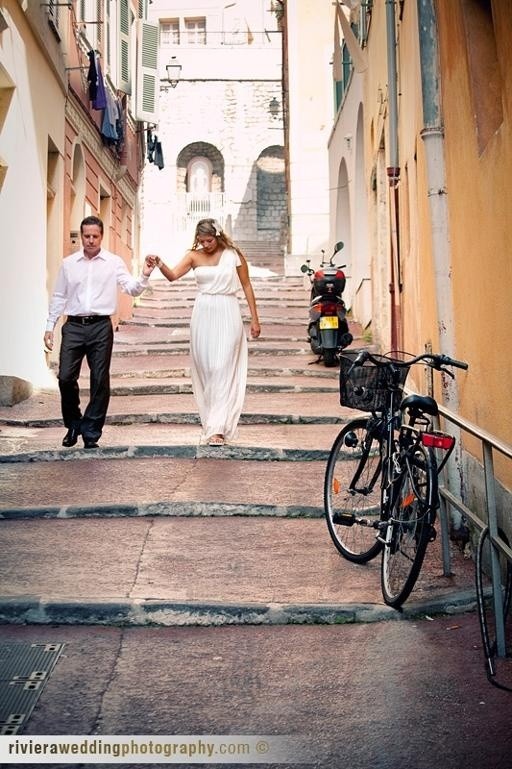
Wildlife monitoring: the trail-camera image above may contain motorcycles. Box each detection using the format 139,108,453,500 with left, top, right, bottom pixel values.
300,241,351,368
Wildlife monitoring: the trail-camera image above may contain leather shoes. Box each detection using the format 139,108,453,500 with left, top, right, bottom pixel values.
62,420,82,447
83,439,100,450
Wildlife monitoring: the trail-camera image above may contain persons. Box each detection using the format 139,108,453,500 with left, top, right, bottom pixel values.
146,218,261,445
44,216,157,448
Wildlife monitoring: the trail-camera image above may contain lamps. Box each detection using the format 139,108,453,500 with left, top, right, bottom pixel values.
268,94,285,122
159,55,183,94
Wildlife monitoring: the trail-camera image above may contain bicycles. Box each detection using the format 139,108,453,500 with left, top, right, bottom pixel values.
324,347,469,608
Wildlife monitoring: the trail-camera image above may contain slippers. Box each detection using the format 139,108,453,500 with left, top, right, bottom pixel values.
205,433,228,448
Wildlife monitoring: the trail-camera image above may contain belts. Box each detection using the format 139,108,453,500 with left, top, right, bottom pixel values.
67,314,110,326
